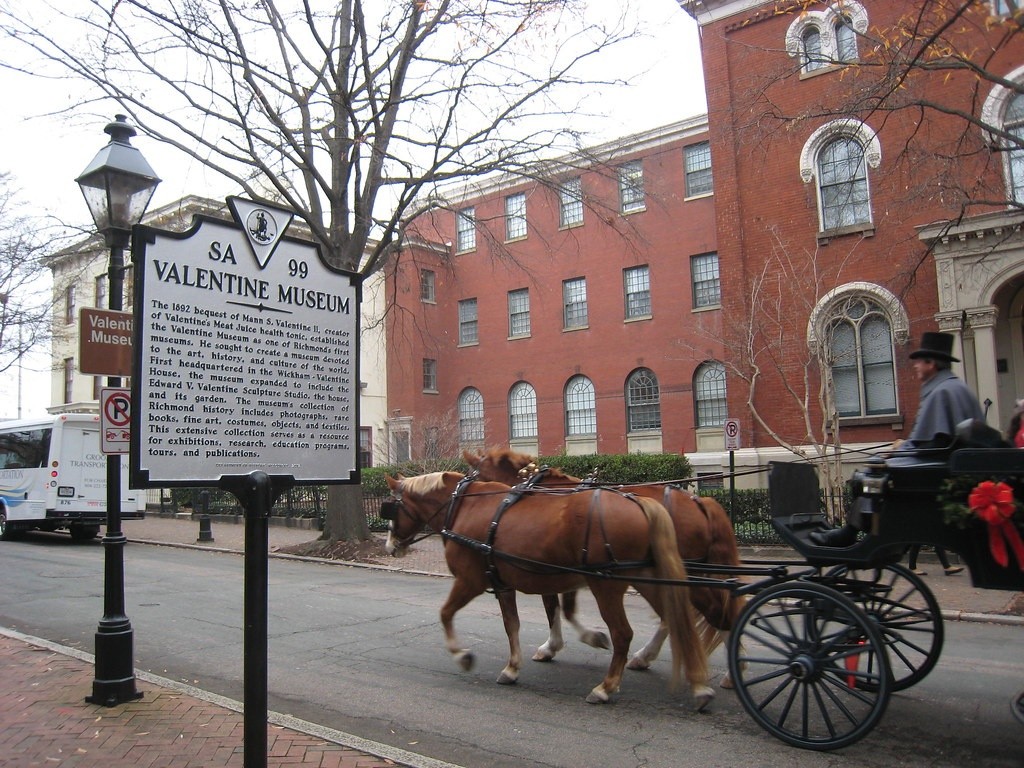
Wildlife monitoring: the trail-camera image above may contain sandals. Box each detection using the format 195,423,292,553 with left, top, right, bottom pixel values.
944,567,965,576
915,570,928,575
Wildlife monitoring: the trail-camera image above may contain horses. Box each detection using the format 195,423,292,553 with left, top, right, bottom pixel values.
383,444,750,712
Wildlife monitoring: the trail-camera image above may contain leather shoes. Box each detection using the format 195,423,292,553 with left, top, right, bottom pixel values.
816,527,828,533
809,529,855,547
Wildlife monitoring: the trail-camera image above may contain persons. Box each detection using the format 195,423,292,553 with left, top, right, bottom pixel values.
807,331,985,550
904,545,965,578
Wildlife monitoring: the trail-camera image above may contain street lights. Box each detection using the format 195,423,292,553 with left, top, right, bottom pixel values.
71,112,161,708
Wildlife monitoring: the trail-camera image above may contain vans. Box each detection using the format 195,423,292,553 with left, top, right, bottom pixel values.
1,406,147,542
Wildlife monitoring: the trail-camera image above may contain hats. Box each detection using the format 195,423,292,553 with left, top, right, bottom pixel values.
1013,399,1024,415
909,331,960,362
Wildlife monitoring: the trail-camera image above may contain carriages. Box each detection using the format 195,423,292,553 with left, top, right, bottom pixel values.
376,316,1024,752
